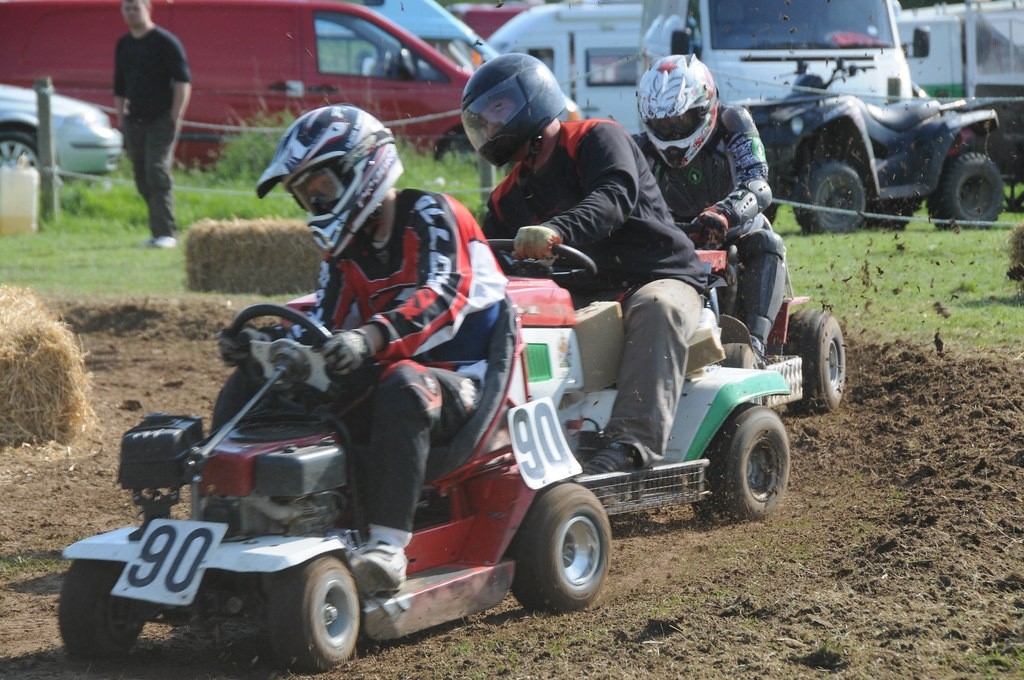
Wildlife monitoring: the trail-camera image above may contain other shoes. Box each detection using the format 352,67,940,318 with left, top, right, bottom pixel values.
580,444,638,478
134,236,177,248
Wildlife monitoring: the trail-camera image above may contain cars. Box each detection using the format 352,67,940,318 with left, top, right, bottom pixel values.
486,3,1024,134
0,78,124,179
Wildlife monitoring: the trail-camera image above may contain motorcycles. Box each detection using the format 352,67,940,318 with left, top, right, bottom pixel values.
732,59,1006,234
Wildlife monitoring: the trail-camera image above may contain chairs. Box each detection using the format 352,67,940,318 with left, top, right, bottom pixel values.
422,293,518,484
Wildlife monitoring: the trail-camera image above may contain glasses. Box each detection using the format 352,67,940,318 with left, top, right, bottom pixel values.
648,109,702,140
290,128,396,215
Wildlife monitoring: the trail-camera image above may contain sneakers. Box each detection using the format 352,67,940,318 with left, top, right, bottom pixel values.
348,541,407,596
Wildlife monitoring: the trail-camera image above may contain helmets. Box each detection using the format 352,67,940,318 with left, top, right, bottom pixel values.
637,54,717,169
460,52,568,167
255,104,404,257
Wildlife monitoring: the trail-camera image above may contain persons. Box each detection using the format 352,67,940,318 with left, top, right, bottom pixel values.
113,0,192,248
461,53,710,477
630,56,788,362
205,105,508,594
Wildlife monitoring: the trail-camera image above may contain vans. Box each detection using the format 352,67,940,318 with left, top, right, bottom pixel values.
312,1,505,82
1,1,474,170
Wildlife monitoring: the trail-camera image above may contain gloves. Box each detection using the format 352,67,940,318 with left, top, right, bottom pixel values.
217,328,269,368
689,206,728,250
323,329,376,375
511,226,562,261
